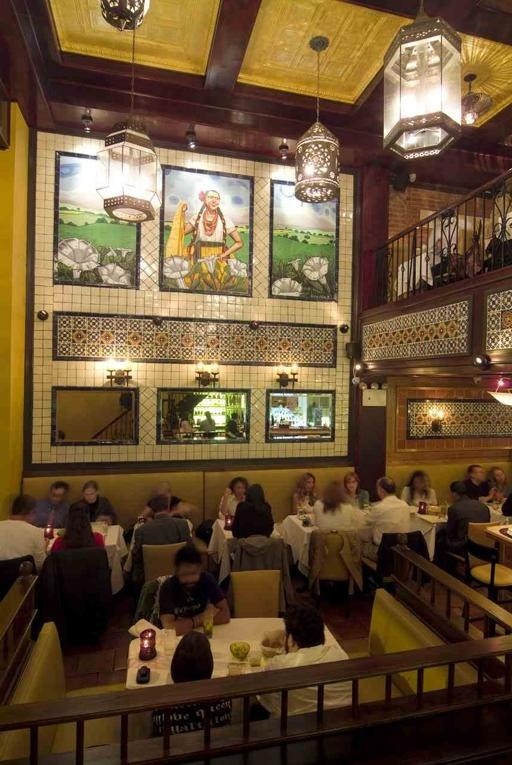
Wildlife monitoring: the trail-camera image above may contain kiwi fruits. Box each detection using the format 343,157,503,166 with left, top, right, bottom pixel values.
271,642,280,649
262,638,270,647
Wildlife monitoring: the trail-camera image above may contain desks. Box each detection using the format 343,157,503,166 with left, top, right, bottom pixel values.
123,616,345,688
44,505,512,598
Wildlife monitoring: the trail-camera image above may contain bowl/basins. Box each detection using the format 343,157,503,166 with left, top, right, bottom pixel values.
229,642,251,660
260,640,286,658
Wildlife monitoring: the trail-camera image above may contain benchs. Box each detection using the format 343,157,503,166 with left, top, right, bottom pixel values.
17,461,512,551
1,622,153,761
346,587,490,709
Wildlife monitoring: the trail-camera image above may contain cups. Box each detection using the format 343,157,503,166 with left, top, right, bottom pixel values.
164,629,177,657
44,525,54,539
201,613,215,640
418,501,426,514
225,514,233,530
139,628,156,661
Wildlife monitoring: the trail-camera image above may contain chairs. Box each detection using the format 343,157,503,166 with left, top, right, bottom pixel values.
0,520,511,646
139,575,171,632
435,228,482,287
231,570,282,617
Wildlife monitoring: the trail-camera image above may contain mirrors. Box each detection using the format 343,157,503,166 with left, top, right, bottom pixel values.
157,386,251,444
264,388,337,443
52,385,139,446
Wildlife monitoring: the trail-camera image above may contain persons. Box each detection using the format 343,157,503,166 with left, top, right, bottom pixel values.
429,206,478,276
158,544,230,635
178,187,244,279
162,409,245,440
293,464,511,553
254,603,353,719
2,478,117,572
131,483,200,580
151,629,232,734
218,476,274,559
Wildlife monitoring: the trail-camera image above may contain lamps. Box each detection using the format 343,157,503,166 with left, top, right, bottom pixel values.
106,360,134,386
94,0,165,223
194,363,222,385
294,35,341,203
80,115,291,161
383,0,463,158
274,365,301,387
431,408,445,431
358,380,389,391
460,72,493,125
